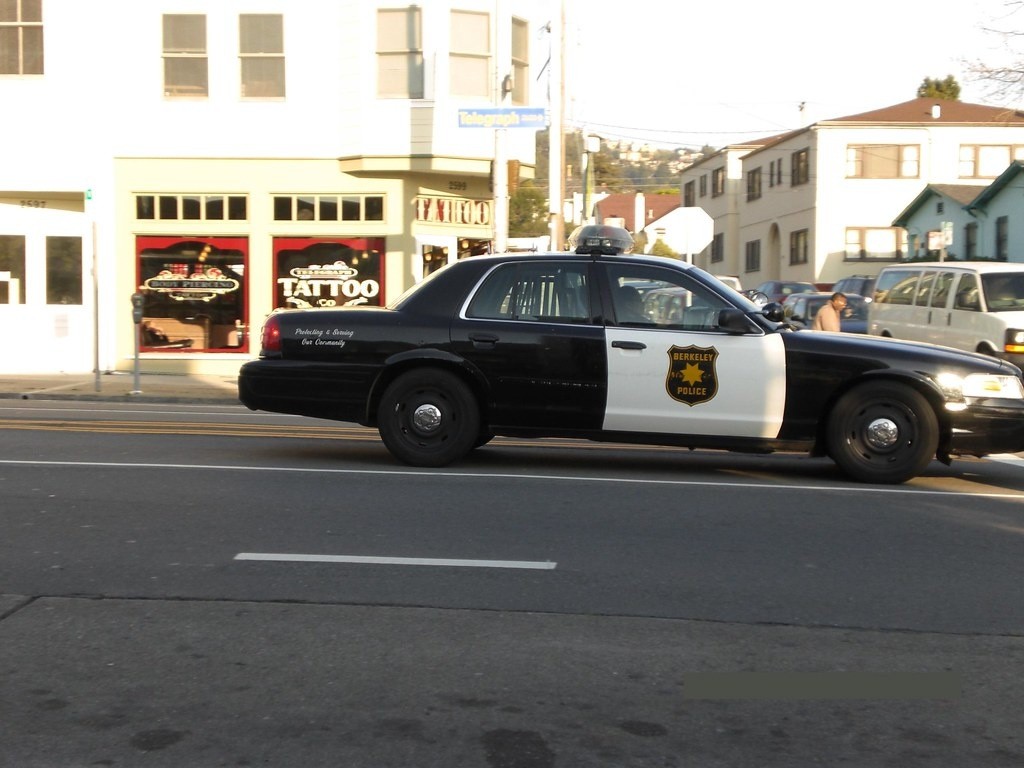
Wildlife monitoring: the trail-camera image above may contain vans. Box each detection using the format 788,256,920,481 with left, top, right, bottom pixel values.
867,259,1024,370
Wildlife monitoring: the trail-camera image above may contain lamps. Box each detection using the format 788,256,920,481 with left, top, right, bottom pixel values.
502,74,515,93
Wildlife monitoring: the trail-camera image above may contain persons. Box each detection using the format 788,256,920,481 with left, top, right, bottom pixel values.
619,286,662,323
812,291,848,331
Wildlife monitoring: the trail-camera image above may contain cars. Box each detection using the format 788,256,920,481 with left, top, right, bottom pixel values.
623,272,878,338
237,223,1024,485
